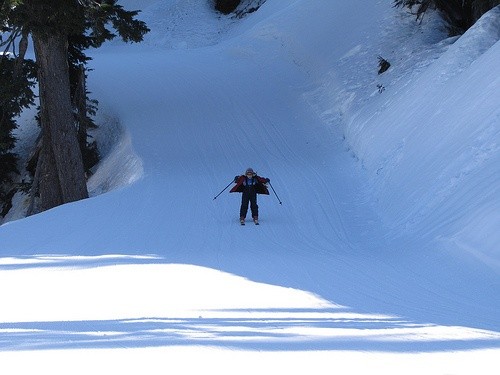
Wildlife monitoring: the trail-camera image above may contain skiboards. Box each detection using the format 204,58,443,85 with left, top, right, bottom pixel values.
241,219,260,226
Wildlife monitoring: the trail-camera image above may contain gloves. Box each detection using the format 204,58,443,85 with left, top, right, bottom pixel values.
266,178,270,182
235,176,239,182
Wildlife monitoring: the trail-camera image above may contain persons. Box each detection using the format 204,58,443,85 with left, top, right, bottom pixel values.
229,168,271,225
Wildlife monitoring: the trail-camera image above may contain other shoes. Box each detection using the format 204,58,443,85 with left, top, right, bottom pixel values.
253,216,258,223
240,216,244,223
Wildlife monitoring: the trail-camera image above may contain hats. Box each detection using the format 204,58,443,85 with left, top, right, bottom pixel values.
246,168,253,176
